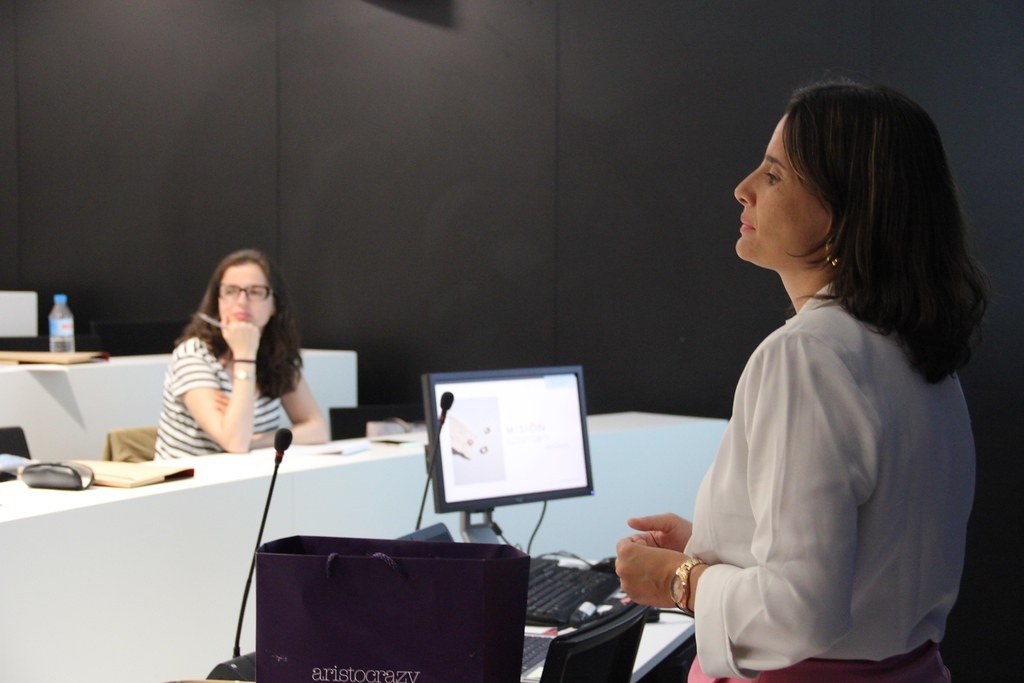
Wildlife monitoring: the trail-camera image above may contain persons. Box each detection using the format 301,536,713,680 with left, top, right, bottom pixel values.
615,82,976,683
153,250,330,461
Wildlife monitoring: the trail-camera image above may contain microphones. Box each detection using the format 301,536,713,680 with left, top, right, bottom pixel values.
207,427,293,683
415,393,454,532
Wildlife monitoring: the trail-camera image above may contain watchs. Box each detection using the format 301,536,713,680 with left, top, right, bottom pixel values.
669,558,705,617
233,369,255,380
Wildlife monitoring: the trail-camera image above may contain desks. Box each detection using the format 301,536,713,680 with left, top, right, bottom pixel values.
0,346,730,683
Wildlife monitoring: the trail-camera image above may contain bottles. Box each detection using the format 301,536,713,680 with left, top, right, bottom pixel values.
48,294,75,352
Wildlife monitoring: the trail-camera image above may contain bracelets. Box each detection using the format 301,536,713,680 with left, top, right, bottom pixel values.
233,359,257,363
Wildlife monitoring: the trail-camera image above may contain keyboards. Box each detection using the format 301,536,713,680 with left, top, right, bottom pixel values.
521,556,621,629
520,634,554,679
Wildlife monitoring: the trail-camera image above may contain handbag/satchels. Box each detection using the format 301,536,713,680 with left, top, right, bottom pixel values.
254,535,532,683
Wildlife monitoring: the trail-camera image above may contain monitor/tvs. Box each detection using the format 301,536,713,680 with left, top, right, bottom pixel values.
420,364,594,545
394,523,456,543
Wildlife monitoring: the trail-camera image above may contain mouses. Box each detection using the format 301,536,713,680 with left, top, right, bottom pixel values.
590,557,619,577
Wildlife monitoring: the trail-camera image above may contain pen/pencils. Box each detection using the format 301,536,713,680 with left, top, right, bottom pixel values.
197,310,221,327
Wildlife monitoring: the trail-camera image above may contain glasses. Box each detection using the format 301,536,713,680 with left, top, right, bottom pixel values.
217,283,272,301
